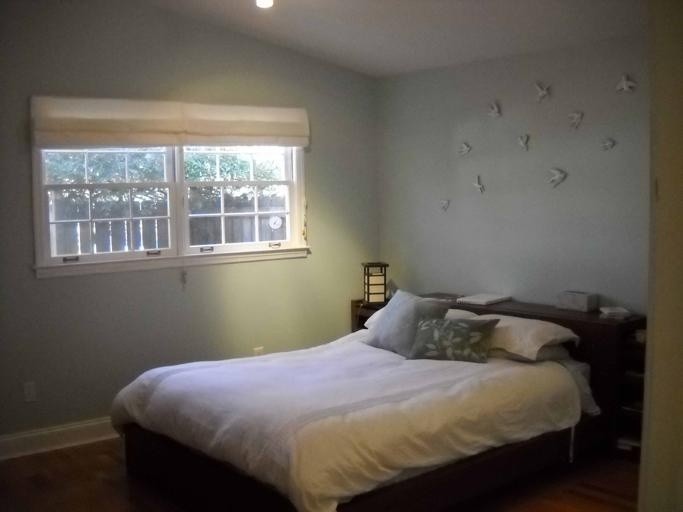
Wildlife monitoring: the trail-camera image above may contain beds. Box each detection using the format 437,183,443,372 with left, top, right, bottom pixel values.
111,293,645,511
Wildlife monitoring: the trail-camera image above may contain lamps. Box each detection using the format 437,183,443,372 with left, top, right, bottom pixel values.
361,260,393,308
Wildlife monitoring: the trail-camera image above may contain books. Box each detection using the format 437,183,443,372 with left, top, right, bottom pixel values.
457,294,511,305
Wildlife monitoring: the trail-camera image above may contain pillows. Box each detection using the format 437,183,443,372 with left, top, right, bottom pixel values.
358,289,581,363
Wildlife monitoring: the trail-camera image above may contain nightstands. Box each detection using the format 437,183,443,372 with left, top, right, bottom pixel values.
349,297,390,332
607,317,644,462
